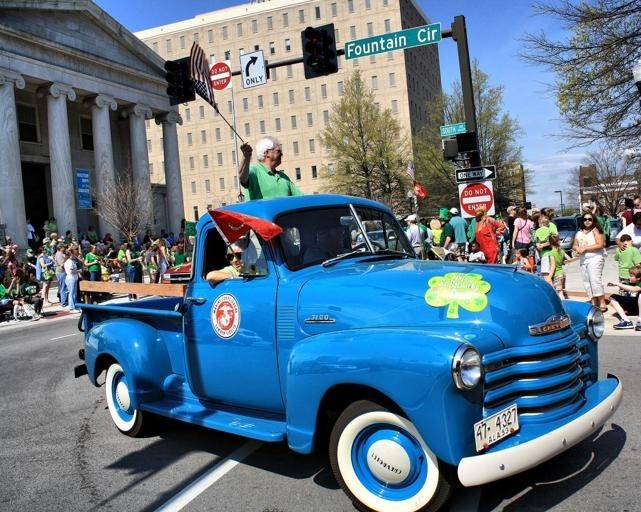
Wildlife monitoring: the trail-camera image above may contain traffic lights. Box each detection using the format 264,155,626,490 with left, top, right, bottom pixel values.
164,56,195,106
301,23,338,82
456,133,479,153
443,141,457,160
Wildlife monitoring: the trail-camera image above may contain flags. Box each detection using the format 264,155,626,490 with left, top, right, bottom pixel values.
188,42,216,111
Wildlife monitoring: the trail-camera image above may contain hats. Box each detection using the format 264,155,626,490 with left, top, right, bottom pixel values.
505,205,517,213
448,207,458,216
403,215,416,221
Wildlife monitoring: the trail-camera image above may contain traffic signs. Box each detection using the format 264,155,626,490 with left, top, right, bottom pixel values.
344,21,441,61
456,164,499,184
239,50,268,89
439,122,465,136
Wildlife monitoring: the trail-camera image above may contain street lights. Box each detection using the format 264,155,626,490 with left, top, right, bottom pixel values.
408,190,414,215
560,203,566,213
555,190,564,217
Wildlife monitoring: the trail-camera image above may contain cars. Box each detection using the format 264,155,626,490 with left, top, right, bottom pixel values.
162,260,193,285
606,218,622,245
361,229,403,253
549,213,581,254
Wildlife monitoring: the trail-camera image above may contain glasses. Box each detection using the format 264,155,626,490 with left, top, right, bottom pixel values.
582,218,592,221
225,251,244,262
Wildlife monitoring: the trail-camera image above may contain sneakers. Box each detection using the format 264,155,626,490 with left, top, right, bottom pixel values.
13,313,44,321
612,318,634,330
635,321,641,331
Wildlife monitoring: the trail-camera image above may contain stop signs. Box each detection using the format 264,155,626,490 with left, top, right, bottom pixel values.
458,181,495,219
206,60,233,92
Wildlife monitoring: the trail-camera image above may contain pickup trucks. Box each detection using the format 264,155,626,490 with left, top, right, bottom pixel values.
77,192,624,511
